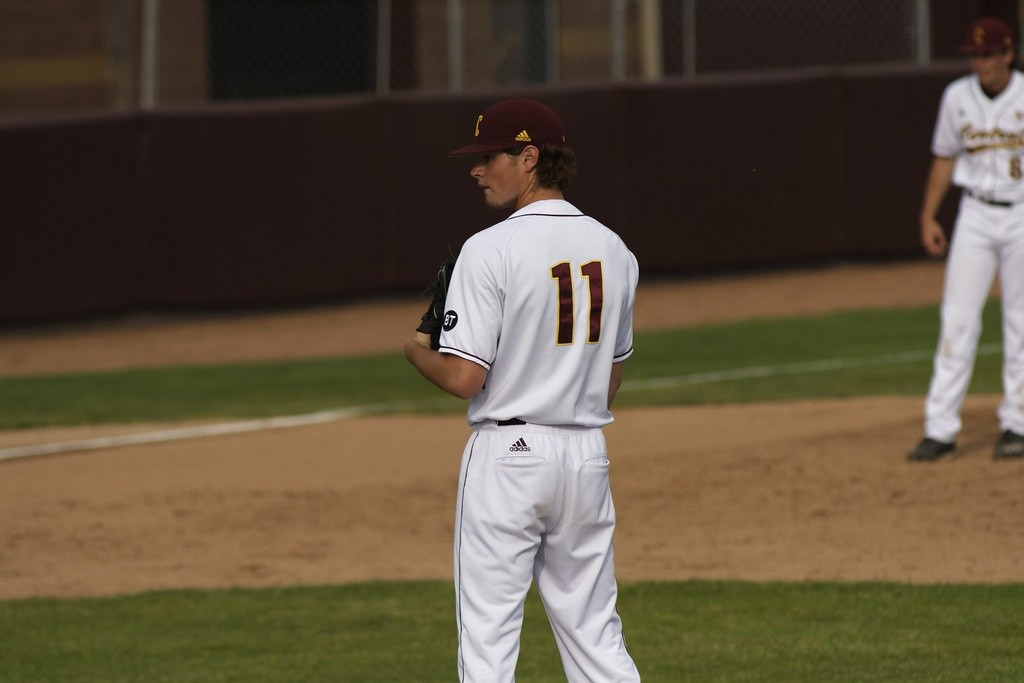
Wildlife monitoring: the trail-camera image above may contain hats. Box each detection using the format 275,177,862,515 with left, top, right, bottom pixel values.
959,18,1012,54
448,99,567,156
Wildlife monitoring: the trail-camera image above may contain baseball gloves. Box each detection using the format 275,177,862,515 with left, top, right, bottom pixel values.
416,249,460,348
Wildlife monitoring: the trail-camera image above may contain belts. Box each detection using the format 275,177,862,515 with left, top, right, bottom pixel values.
984,200,1011,206
495,418,526,427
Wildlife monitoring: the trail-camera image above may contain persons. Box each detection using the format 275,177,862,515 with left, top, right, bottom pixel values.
904,18,1024,460
404,97,641,683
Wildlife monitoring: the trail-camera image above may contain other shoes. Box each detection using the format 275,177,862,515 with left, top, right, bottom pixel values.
910,438,956,460
995,432,1024,457
